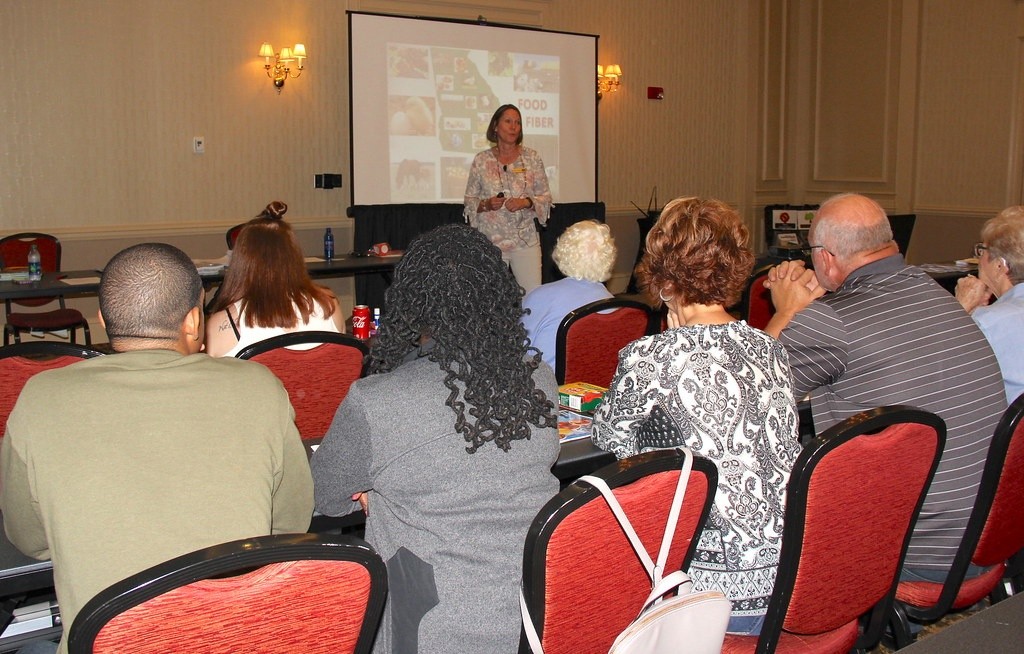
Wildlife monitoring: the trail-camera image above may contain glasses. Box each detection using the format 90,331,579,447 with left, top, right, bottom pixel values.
974,243,989,257
800,246,837,258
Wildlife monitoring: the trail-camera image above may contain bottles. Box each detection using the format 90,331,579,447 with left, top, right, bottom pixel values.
28,245,41,281
324,228,334,260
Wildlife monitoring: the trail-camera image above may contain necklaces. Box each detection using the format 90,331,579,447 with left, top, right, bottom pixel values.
496,145,526,199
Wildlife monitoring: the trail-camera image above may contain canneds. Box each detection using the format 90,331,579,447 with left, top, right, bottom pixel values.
352,305,370,339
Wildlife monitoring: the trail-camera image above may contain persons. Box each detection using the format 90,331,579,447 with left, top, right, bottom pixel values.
462,104,555,294
761,193,1009,651
954,206,1024,407
590,195,804,636
519,220,617,375
0,244,316,654
309,225,561,654
204,202,347,358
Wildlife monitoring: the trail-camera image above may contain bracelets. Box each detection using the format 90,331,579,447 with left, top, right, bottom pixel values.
481,199,491,212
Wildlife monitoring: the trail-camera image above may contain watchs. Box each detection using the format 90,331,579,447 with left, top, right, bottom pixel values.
526,197,533,208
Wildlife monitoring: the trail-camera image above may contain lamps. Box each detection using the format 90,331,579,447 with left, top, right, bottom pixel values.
598,63,622,97
257,42,307,87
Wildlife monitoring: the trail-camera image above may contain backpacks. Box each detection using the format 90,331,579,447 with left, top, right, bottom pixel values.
518,446,732,654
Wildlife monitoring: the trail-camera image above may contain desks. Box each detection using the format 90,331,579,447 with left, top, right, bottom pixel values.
1,391,809,595
0,250,402,300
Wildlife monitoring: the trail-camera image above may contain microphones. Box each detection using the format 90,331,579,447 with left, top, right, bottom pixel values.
503,165,507,171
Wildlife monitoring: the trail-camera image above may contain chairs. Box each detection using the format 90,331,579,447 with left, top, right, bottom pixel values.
225,223,249,253
0,231,90,345
0,260,1023,654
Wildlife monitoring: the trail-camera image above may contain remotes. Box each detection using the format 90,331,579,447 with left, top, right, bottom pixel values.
497,192,504,198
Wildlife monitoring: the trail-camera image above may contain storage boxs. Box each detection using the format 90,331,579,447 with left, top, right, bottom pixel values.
558,380,610,412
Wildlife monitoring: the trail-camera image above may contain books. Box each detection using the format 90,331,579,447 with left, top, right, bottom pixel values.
195,262,224,275
12,587,61,622
